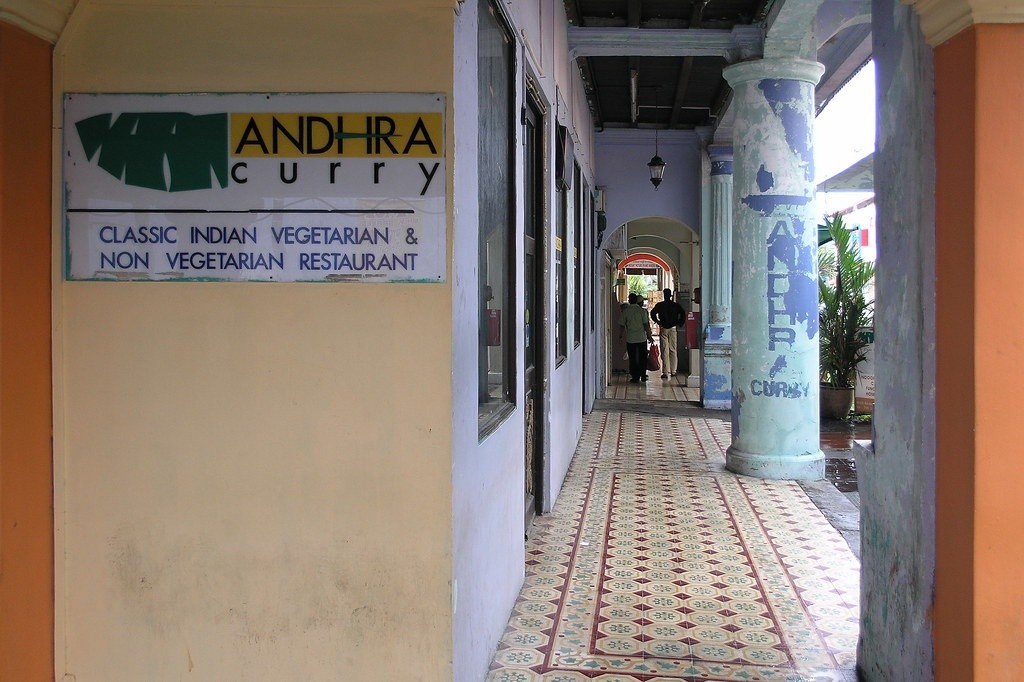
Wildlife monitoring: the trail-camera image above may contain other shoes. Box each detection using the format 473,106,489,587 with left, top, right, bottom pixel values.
629,375,649,384
661,371,677,379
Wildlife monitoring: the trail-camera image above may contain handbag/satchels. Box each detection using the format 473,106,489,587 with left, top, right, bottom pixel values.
646,342,660,371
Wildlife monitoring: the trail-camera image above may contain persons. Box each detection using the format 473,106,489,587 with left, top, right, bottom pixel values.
618,293,655,383
651,287,685,378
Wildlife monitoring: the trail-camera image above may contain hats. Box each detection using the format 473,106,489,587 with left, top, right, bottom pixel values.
638,295,648,304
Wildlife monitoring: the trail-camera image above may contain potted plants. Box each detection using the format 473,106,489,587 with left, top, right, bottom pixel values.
818,212,875,422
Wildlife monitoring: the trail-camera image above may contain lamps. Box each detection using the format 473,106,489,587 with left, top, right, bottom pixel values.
647,106,667,192
